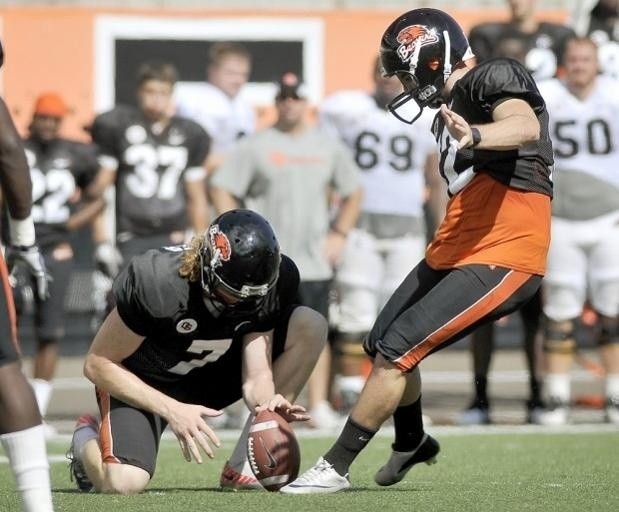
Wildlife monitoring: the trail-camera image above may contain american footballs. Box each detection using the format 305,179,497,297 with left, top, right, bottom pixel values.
246,409,299,493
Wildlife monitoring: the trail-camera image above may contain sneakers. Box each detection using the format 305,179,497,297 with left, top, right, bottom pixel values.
371,434,440,487
69,412,99,491
219,461,266,490
278,454,351,496
452,389,617,428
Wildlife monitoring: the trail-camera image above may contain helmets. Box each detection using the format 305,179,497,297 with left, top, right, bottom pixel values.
195,208,281,317
379,6,474,109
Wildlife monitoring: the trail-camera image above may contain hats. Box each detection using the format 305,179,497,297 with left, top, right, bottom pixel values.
34,92,72,117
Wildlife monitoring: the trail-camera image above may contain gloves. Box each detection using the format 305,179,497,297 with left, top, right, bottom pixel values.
7,241,54,314
276,71,307,104
